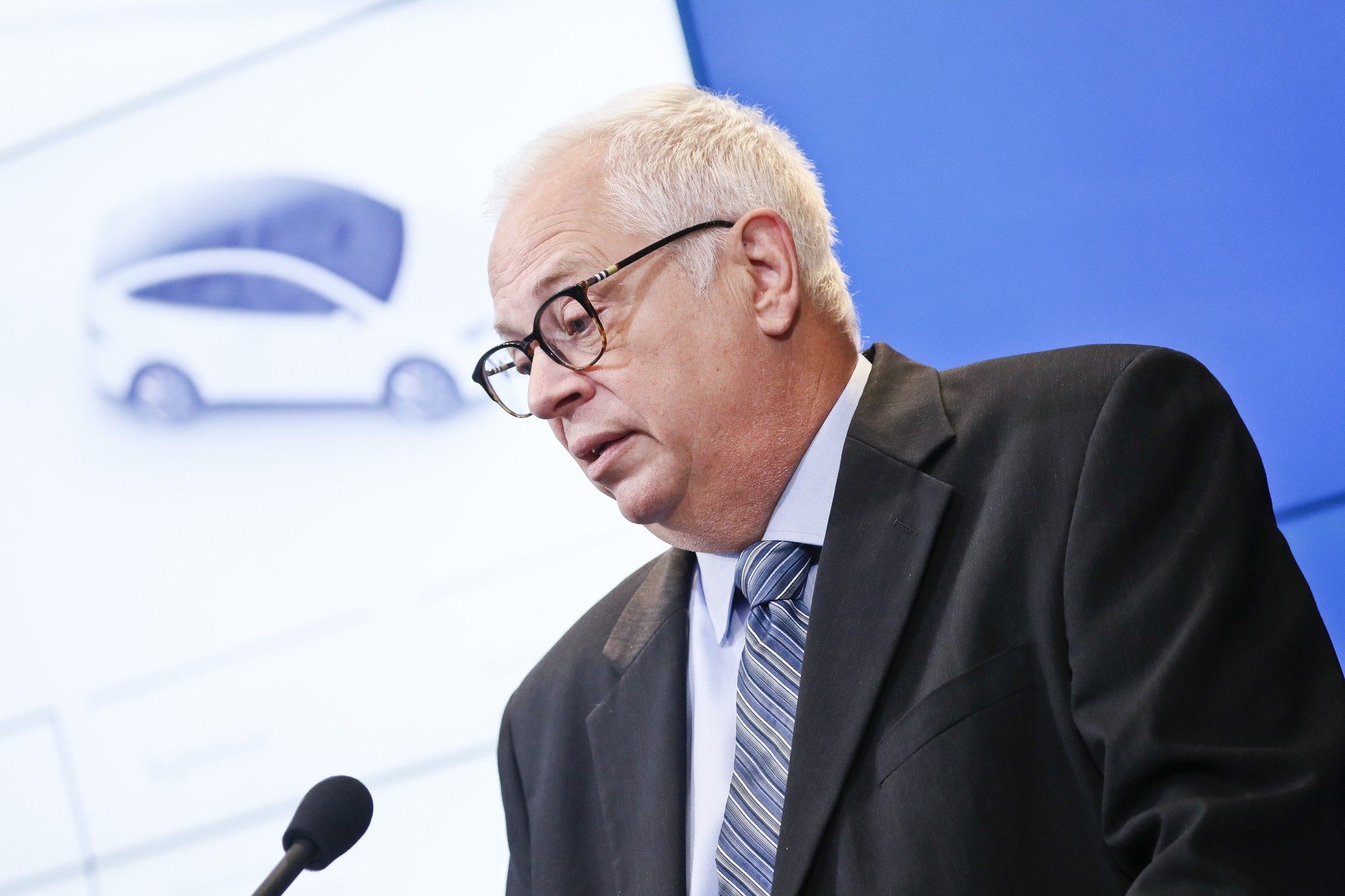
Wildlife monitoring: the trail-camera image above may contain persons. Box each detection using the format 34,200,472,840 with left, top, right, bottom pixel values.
470,86,1345,896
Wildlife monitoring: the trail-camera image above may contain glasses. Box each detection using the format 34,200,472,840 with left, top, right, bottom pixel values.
472,219,734,418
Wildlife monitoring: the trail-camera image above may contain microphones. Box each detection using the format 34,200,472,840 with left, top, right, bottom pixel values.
250,776,374,896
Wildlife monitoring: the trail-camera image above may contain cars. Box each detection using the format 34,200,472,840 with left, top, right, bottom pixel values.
82,167,482,419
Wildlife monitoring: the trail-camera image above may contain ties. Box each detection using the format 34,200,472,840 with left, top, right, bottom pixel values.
717,541,814,896
